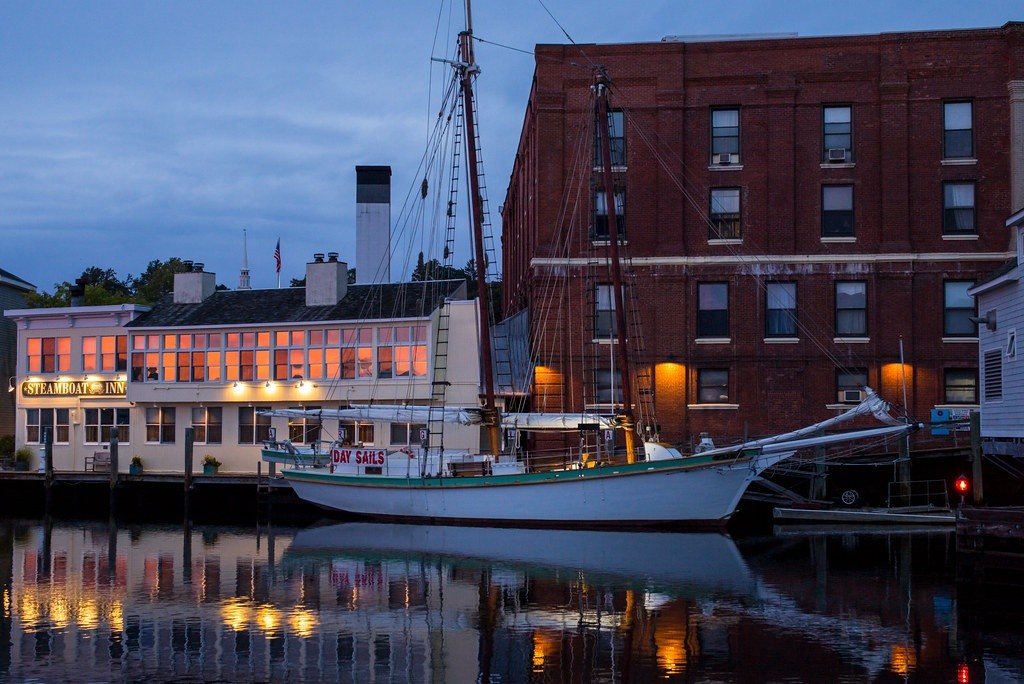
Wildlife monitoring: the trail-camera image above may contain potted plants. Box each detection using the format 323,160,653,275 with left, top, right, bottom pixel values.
15,449,32,471
129,455,144,474
200,454,222,476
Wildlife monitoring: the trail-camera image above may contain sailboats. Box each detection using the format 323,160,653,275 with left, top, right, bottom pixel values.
281,520,963,637
259,0,925,533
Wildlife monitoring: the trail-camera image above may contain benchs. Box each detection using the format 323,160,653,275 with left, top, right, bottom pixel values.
447,461,492,477
84,451,112,472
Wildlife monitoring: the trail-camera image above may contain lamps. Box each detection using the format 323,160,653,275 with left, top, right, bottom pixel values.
300,379,304,387
56,375,60,381
84,373,88,380
265,380,271,387
956,475,970,496
233,380,239,388
8,376,17,393
664,342,678,362
26,375,29,381
116,372,121,381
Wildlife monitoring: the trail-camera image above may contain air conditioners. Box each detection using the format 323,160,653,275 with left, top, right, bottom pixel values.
828,148,846,162
717,153,731,164
842,390,863,403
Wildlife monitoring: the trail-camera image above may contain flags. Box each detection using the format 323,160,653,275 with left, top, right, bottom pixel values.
274,241,281,272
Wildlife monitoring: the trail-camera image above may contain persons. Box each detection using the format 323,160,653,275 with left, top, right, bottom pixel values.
420,431,425,438
138,367,159,382
